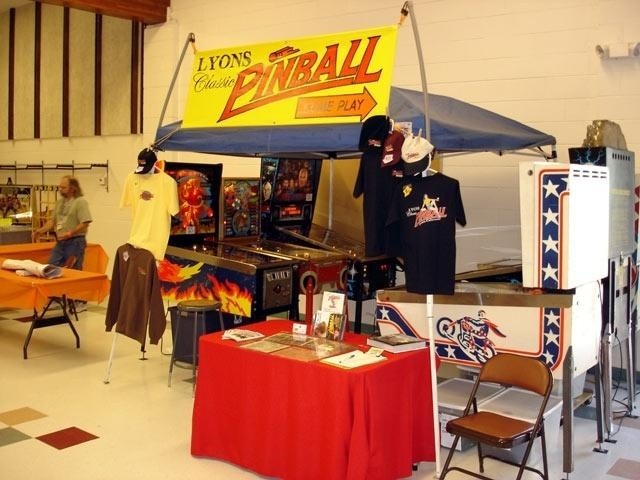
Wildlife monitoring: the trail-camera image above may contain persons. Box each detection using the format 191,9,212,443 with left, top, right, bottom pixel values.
32,176,93,313
262,181,271,205
462,309,507,349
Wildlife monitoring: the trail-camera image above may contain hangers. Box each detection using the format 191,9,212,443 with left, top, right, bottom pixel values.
148,163,164,175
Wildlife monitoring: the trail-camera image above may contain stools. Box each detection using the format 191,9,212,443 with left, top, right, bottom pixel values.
168,299,223,396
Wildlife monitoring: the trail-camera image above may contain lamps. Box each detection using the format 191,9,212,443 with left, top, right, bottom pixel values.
596,41,640,60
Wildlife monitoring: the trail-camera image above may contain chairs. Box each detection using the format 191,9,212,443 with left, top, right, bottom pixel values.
439,353,553,480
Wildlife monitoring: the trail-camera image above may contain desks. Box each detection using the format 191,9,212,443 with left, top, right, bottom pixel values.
0,240,111,359
191,319,442,480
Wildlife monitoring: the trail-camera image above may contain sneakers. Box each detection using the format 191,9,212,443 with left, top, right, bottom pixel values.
45,300,62,310
72,302,88,313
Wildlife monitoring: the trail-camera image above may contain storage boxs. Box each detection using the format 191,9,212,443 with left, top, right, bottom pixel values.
550,369,586,399
431,376,506,453
474,386,564,466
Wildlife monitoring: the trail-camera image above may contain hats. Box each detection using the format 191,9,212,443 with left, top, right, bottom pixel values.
359,115,434,175
134,148,157,173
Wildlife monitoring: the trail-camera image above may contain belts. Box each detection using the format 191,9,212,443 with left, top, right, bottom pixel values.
66,235,85,239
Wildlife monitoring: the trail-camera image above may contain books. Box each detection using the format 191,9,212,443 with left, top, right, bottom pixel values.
221,325,266,342
312,290,428,370
